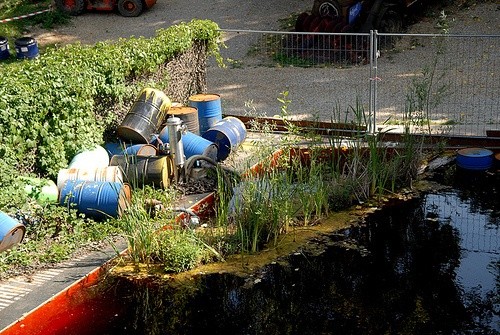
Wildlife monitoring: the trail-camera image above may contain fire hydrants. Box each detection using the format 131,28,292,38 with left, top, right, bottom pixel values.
162,114,189,181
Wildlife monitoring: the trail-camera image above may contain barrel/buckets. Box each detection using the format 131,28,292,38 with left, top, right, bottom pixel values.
456,148,496,171
0,209,25,255
18,89,249,215
14,35,38,61
0,35,10,63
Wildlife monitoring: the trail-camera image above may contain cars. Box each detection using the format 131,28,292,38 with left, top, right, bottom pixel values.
55,0,158,17
